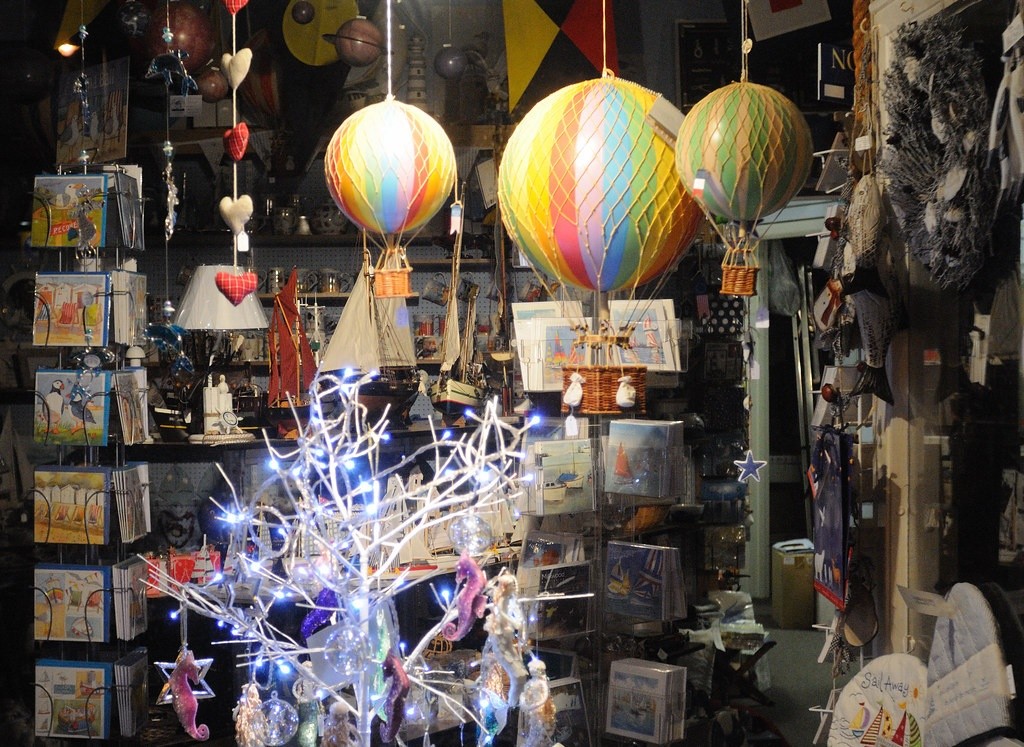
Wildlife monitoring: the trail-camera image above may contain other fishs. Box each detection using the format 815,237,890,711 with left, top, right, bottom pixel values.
847,174,906,407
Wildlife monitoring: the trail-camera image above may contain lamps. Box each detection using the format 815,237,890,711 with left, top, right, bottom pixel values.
173,265,269,434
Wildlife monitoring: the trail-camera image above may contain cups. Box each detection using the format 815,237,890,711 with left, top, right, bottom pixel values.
413,314,502,358
246,266,267,294
244,214,267,236
268,266,290,294
272,207,299,235
485,272,514,302
456,272,481,303
517,275,548,301
231,306,352,362
318,269,351,292
294,267,318,293
422,273,449,307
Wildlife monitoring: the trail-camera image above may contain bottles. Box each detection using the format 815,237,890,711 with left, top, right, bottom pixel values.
288,194,303,229
144,542,222,595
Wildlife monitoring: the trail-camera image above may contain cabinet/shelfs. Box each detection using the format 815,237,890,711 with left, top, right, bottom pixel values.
32,165,152,747
518,414,689,747
220,234,498,368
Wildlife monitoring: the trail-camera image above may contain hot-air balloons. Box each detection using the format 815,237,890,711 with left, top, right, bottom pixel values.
324,95,457,299
674,68,815,297
495,69,704,416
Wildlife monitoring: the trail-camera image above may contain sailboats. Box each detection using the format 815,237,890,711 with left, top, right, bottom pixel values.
427,253,502,418
256,265,338,440
308,224,426,431
